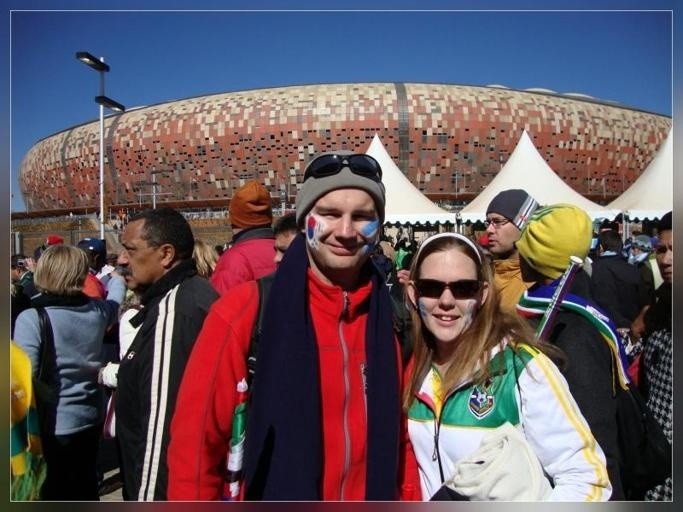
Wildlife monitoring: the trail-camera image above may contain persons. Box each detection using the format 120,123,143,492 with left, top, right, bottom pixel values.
11,244,42,300
614,215,629,258
399,232,612,503
273,214,299,266
630,234,661,303
597,220,611,232
107,251,126,313
508,203,630,502
482,189,539,325
14,245,125,499
112,208,219,503
192,237,218,280
638,211,674,503
77,238,108,298
592,230,650,341
208,180,275,286
378,225,411,304
167,150,425,502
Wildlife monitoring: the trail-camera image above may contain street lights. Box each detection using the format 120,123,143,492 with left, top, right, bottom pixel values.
72,48,124,239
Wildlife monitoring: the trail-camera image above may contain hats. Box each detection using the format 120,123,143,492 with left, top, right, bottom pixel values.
480,235,490,246
485,189,539,232
631,234,653,252
77,237,107,254
46,234,65,245
294,149,386,227
228,178,273,229
514,203,593,280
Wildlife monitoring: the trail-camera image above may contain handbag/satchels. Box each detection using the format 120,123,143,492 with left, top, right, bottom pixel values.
221,400,252,502
25,342,63,439
616,383,672,501
626,355,641,386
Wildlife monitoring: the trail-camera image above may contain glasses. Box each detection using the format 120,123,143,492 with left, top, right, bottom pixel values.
415,278,480,298
481,215,511,227
304,153,383,184
